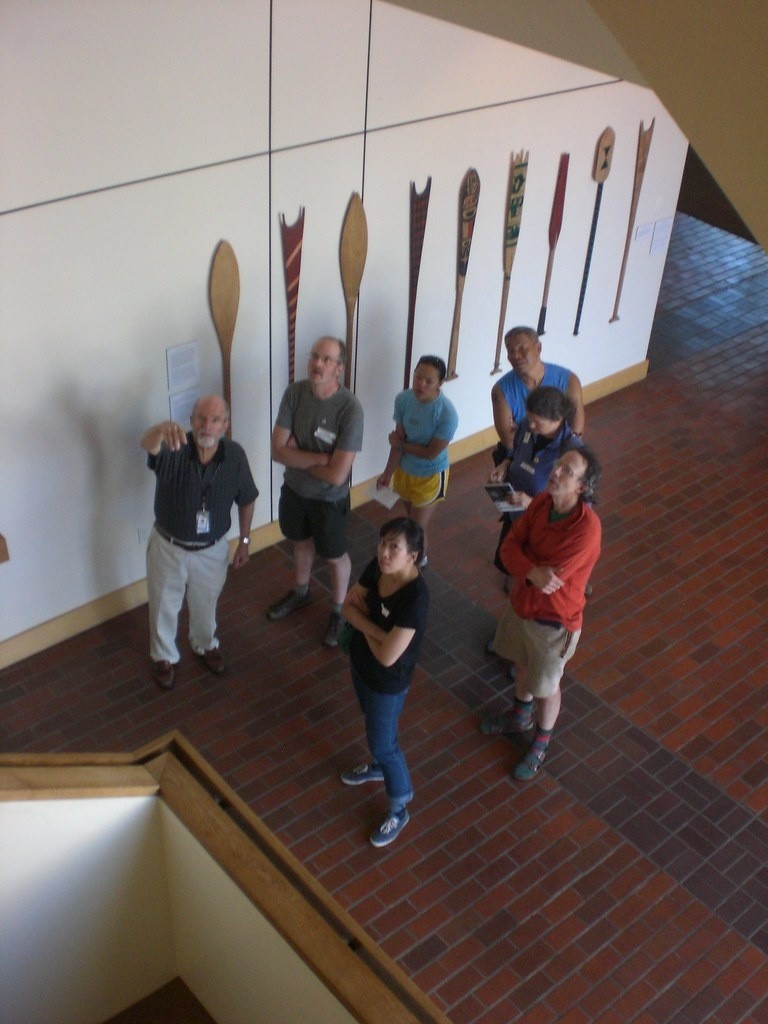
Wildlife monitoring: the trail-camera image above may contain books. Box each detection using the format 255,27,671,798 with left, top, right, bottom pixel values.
483,483,524,512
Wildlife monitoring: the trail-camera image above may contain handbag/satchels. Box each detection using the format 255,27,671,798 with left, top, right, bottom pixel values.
337,619,356,653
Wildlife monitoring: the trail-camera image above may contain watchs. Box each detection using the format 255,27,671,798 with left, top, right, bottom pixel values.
239,536,250,545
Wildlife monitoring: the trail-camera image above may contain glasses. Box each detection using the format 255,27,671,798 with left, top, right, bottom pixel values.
553,458,587,484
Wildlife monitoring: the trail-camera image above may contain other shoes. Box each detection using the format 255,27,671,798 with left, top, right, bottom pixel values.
484,636,496,654
505,658,516,679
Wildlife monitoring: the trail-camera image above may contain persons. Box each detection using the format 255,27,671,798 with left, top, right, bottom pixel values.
140,394,259,689
265,336,364,649
376,356,459,570
482,327,603,780
340,515,429,847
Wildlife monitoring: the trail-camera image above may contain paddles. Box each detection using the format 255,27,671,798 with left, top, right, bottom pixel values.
536,152,571,335
609,116,656,324
444,168,482,382
572,126,616,336
403,175,433,392
489,150,530,377
278,205,308,387
338,192,370,391
206,237,242,442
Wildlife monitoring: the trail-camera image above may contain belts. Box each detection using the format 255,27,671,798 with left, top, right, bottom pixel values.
535,616,564,629
153,521,221,551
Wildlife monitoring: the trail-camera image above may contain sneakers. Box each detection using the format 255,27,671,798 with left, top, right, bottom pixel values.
341,762,385,784
370,804,409,847
480,711,533,733
513,746,550,780
323,610,344,646
267,590,312,619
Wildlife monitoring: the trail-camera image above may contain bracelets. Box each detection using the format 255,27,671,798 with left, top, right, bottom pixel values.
396,440,404,453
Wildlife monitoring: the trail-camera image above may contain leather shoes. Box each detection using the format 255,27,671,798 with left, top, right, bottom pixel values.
152,659,175,688
205,646,225,673
